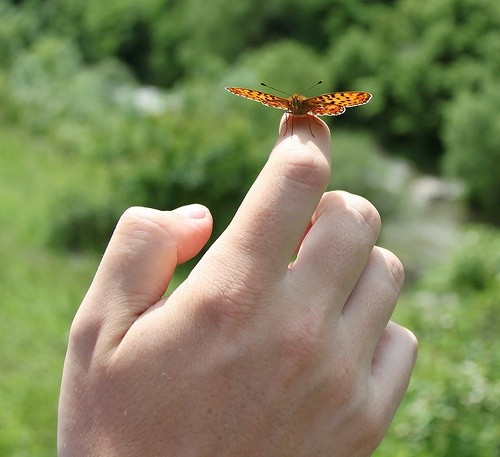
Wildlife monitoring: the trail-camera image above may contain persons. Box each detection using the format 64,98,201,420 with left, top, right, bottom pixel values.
58,113,418,457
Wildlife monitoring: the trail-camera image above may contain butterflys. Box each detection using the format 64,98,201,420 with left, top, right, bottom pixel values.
224,79,372,138
